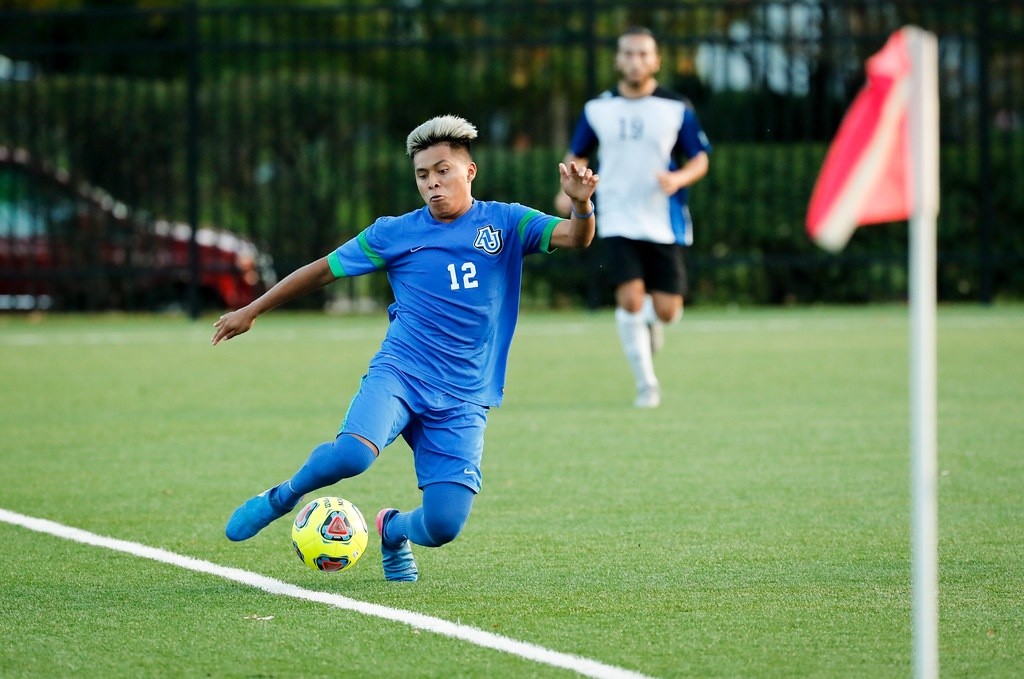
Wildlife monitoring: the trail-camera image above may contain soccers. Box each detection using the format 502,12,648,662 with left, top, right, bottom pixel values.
291,493,371,572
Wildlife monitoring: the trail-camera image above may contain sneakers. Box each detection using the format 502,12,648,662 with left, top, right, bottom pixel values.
635,386,662,409
648,322,664,352
375,507,419,582
226,480,305,541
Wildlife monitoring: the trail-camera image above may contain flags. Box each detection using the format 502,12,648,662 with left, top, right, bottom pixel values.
805,26,910,257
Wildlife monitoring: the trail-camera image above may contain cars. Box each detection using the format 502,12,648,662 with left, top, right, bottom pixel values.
0,145,265,313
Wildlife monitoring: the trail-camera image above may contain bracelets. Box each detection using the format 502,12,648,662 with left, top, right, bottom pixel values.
571,200,594,219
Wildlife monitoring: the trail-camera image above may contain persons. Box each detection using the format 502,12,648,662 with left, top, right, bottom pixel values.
554,27,711,409
210,115,600,584
